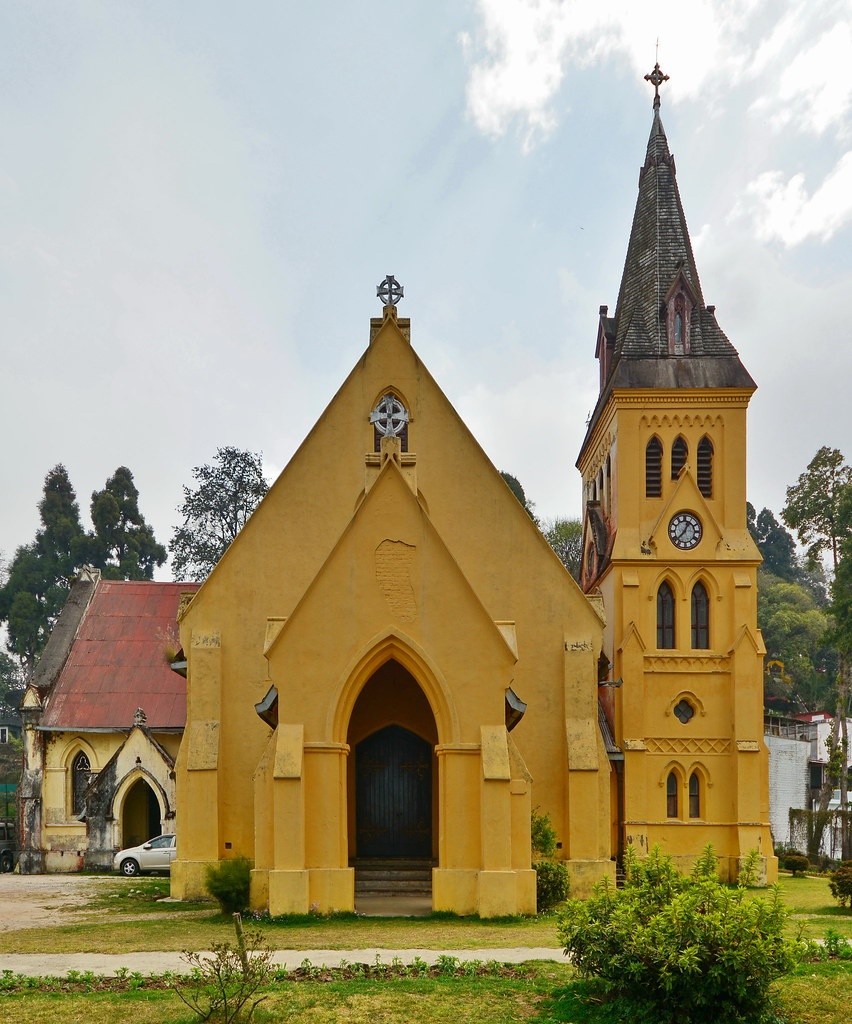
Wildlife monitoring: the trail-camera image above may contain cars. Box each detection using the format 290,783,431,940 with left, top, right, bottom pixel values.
113,833,177,877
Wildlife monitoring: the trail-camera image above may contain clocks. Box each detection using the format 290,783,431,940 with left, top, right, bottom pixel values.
668,511,703,550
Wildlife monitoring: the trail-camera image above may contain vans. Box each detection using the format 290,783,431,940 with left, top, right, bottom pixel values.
0,823,14,873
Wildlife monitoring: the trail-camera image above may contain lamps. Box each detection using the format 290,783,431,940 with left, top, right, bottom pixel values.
135,756,142,771
